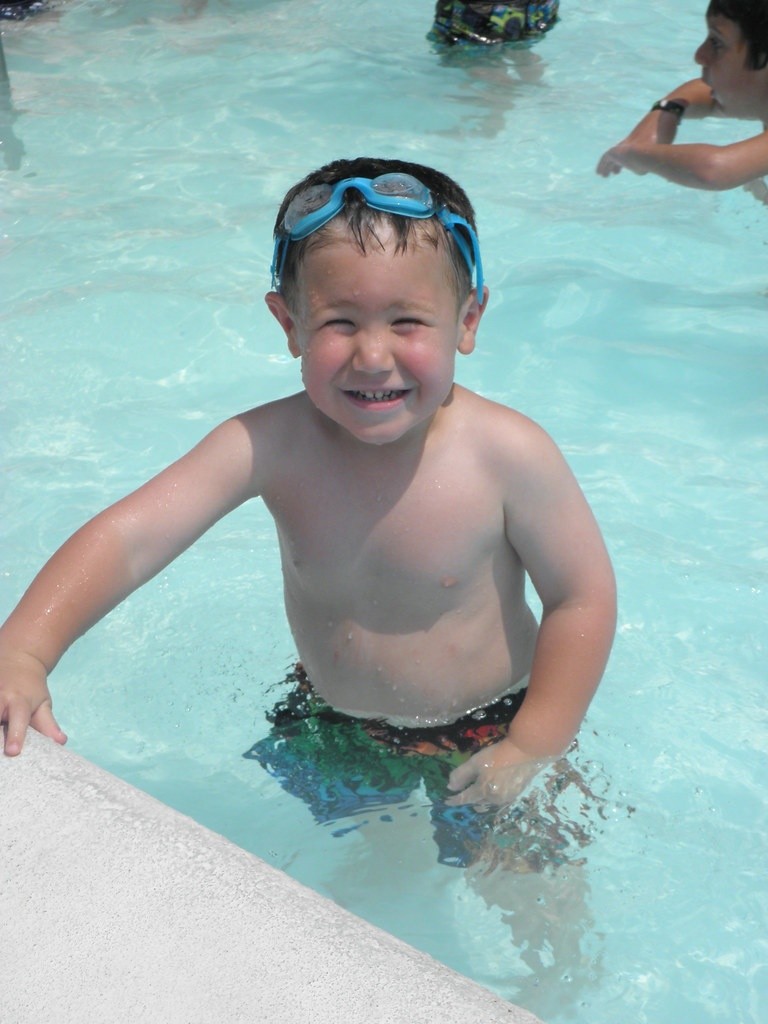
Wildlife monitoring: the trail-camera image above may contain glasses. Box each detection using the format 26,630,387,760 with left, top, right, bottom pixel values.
270,173,484,306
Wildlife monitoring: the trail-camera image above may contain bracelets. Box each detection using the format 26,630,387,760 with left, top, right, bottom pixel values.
652,99,684,118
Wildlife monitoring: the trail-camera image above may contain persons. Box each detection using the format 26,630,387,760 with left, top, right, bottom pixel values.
597,0,768,204
426,0,559,69
0,159,617,812
